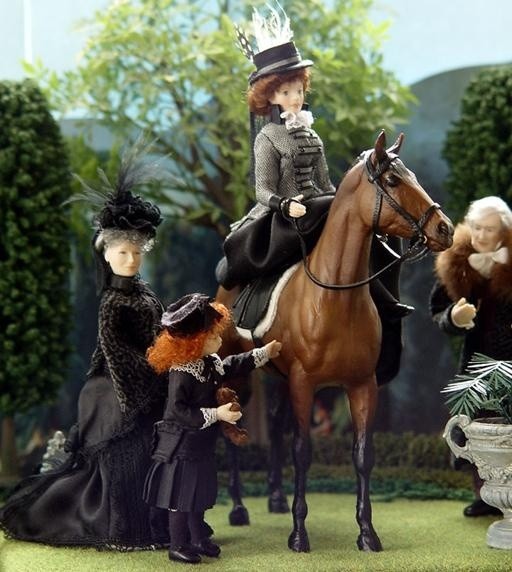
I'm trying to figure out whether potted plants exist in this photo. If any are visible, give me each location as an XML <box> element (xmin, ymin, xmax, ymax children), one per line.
<box><xmin>442</xmin><ymin>351</ymin><xmax>512</xmax><ymax>549</ymax></box>
